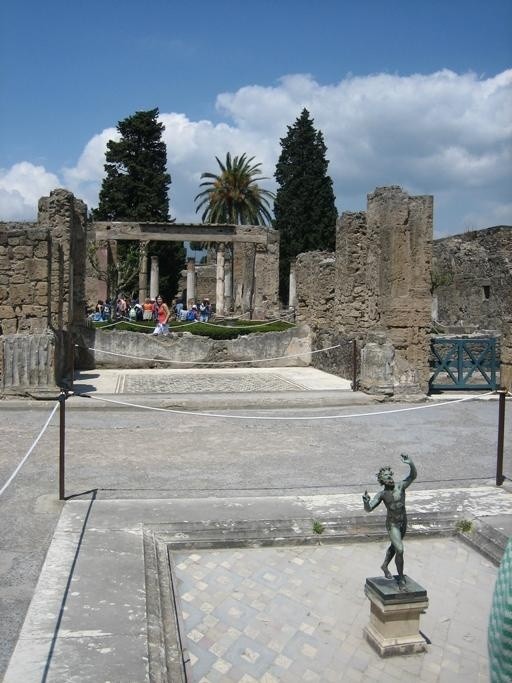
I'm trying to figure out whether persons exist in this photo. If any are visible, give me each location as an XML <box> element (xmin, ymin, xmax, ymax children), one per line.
<box><xmin>361</xmin><ymin>451</ymin><xmax>419</xmax><ymax>592</ymax></box>
<box><xmin>91</xmin><ymin>294</ymin><xmax>215</xmax><ymax>335</ymax></box>
